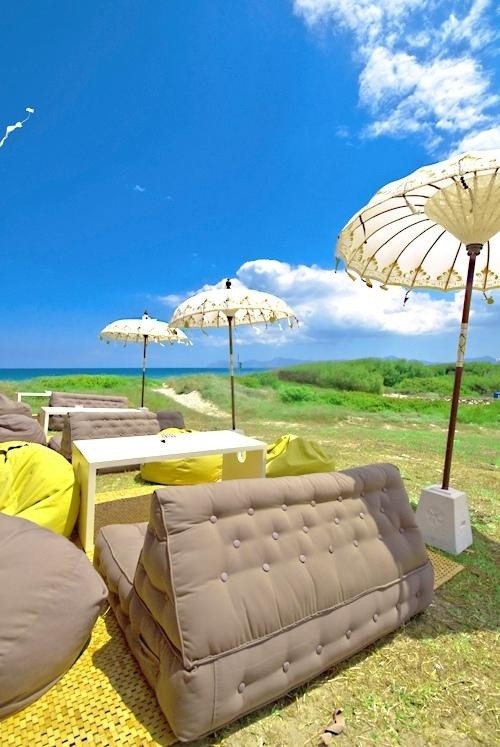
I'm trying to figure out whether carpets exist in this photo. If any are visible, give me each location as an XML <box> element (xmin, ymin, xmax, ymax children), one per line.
<box><xmin>0</xmin><ymin>483</ymin><xmax>465</xmax><ymax>747</ymax></box>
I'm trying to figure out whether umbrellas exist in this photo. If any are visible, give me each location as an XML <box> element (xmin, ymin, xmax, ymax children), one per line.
<box><xmin>337</xmin><ymin>149</ymin><xmax>499</xmax><ymax>490</ymax></box>
<box><xmin>168</xmin><ymin>278</ymin><xmax>298</xmax><ymax>430</ymax></box>
<box><xmin>98</xmin><ymin>309</ymin><xmax>191</xmax><ymax>407</ymax></box>
